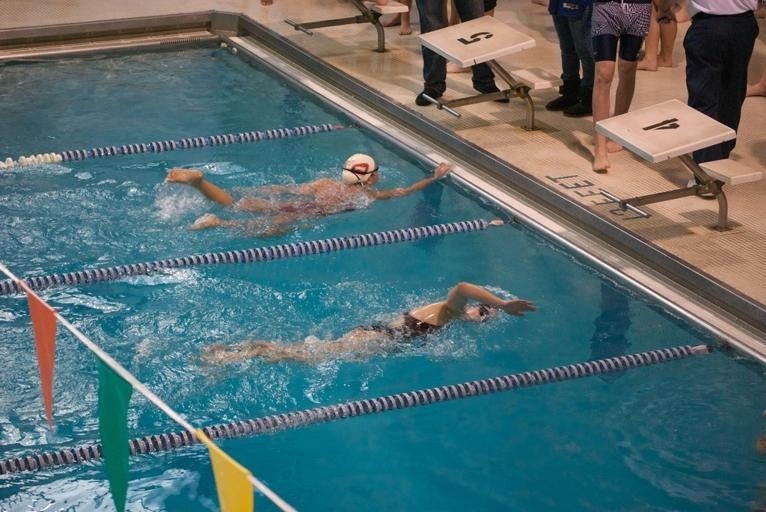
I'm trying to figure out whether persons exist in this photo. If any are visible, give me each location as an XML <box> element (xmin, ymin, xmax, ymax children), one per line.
<box><xmin>591</xmin><ymin>0</ymin><xmax>654</xmax><ymax>174</ymax></box>
<box><xmin>202</xmin><ymin>281</ymin><xmax>538</xmax><ymax>365</ymax></box>
<box><xmin>683</xmin><ymin>0</ymin><xmax>762</xmax><ymax>197</ymax></box>
<box><xmin>383</xmin><ymin>0</ymin><xmax>675</xmax><ymax>118</ymax></box>
<box><xmin>166</xmin><ymin>152</ymin><xmax>452</xmax><ymax>237</ymax></box>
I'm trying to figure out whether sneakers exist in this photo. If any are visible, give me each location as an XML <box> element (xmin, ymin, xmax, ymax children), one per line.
<box><xmin>688</xmin><ymin>180</ymin><xmax>715</xmax><ymax>196</ymax></box>
<box><xmin>416</xmin><ymin>89</ymin><xmax>437</xmax><ymax>105</ymax></box>
<box><xmin>473</xmin><ymin>84</ymin><xmax>510</xmax><ymax>102</ymax></box>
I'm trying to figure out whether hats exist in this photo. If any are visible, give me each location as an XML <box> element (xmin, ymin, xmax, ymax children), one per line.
<box><xmin>342</xmin><ymin>154</ymin><xmax>375</xmax><ymax>185</ymax></box>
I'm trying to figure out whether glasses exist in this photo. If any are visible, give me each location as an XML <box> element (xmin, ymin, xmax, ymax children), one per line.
<box><xmin>478</xmin><ymin>306</ymin><xmax>490</xmax><ymax>323</ymax></box>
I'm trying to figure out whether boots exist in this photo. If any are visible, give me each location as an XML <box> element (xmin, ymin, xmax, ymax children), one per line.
<box><xmin>546</xmin><ymin>79</ymin><xmax>593</xmax><ymax>117</ymax></box>
